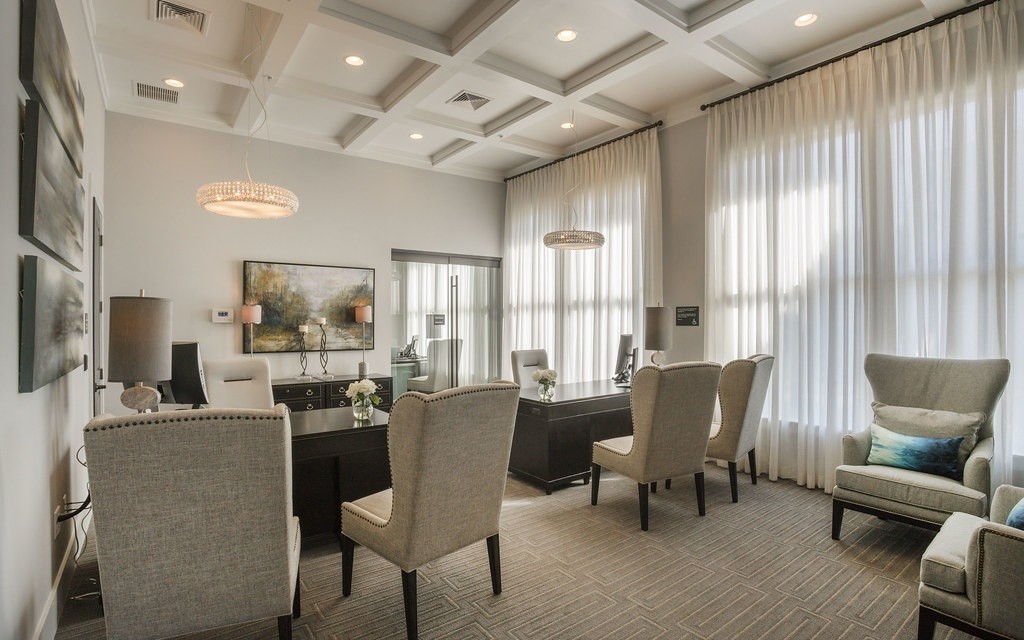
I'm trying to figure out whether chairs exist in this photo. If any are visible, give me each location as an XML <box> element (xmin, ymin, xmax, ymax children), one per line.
<box><xmin>84</xmin><ymin>339</ymin><xmax>1024</xmax><ymax>640</ymax></box>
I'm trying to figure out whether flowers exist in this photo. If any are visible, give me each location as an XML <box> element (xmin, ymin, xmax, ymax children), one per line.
<box><xmin>346</xmin><ymin>379</ymin><xmax>383</xmax><ymax>406</ymax></box>
<box><xmin>533</xmin><ymin>369</ymin><xmax>557</xmax><ymax>391</ymax></box>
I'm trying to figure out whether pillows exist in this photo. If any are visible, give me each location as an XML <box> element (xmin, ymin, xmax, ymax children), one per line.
<box><xmin>1006</xmin><ymin>497</ymin><xmax>1024</xmax><ymax>531</ymax></box>
<box><xmin>865</xmin><ymin>423</ymin><xmax>964</xmax><ymax>480</ymax></box>
<box><xmin>871</xmin><ymin>401</ymin><xmax>988</xmax><ymax>467</ymax></box>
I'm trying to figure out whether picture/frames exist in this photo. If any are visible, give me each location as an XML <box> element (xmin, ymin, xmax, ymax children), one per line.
<box><xmin>243</xmin><ymin>260</ymin><xmax>375</xmax><ymax>354</ymax></box>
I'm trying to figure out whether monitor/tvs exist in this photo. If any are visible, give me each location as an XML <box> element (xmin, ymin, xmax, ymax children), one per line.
<box><xmin>615</xmin><ymin>335</ymin><xmax>638</xmax><ymax>388</ymax></box>
<box><xmin>123</xmin><ymin>341</ymin><xmax>209</xmax><ymax>404</ymax></box>
<box><xmin>409</xmin><ymin>334</ymin><xmax>420</xmax><ymax>358</ymax></box>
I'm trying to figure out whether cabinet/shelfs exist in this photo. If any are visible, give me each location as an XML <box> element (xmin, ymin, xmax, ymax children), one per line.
<box><xmin>314</xmin><ymin>373</ymin><xmax>393</xmax><ymax>410</ymax></box>
<box><xmin>271</xmin><ymin>376</ymin><xmax>325</xmax><ymax>412</ymax></box>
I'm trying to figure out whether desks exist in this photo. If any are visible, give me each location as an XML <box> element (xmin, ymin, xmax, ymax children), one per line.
<box><xmin>392</xmin><ymin>356</ymin><xmax>427</xmax><ymax>377</ymax></box>
<box><xmin>507</xmin><ymin>380</ymin><xmax>634</xmax><ymax>495</ymax></box>
<box><xmin>290</xmin><ymin>406</ymin><xmax>390</xmax><ymax>536</ymax></box>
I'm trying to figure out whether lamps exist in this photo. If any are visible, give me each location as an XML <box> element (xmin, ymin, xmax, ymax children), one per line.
<box><xmin>355</xmin><ymin>306</ymin><xmax>373</xmax><ymax>375</ymax></box>
<box><xmin>543</xmin><ymin>108</ymin><xmax>605</xmax><ymax>250</ymax></box>
<box><xmin>645</xmin><ymin>302</ymin><xmax>672</xmax><ymax>367</ymax></box>
<box><xmin>107</xmin><ymin>288</ymin><xmax>172</xmax><ymax>414</ymax></box>
<box><xmin>242</xmin><ymin>305</ymin><xmax>262</xmax><ymax>357</ymax></box>
<box><xmin>195</xmin><ymin>0</ymin><xmax>299</xmax><ymax>218</ymax></box>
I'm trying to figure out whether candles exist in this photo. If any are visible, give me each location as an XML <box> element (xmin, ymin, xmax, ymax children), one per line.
<box><xmin>299</xmin><ymin>326</ymin><xmax>308</xmax><ymax>333</ymax></box>
<box><xmin>317</xmin><ymin>317</ymin><xmax>326</xmax><ymax>325</ymax></box>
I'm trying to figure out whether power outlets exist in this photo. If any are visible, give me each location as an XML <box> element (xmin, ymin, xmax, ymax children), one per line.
<box><xmin>53</xmin><ymin>504</ymin><xmax>63</xmax><ymax>540</ymax></box>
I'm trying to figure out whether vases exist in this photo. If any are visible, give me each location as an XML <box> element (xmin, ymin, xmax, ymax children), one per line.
<box><xmin>538</xmin><ymin>385</ymin><xmax>554</xmax><ymax>399</ymax></box>
<box><xmin>352</xmin><ymin>398</ymin><xmax>373</xmax><ymax>419</ymax></box>
